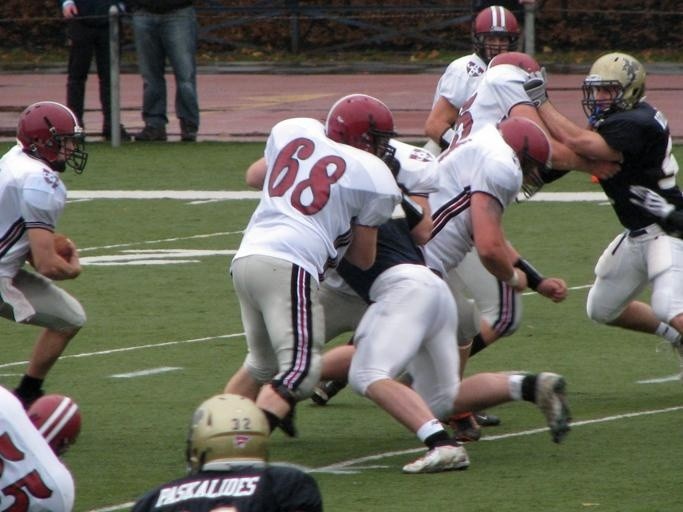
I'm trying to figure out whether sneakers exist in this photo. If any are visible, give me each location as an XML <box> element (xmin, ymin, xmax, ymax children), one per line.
<box><xmin>537</xmin><ymin>371</ymin><xmax>571</xmax><ymax>444</ymax></box>
<box><xmin>403</xmin><ymin>411</ymin><xmax>502</xmax><ymax>473</ymax></box>
<box><xmin>310</xmin><ymin>379</ymin><xmax>345</xmax><ymax>405</ymax></box>
<box><xmin>102</xmin><ymin>124</ymin><xmax>197</xmax><ymax>141</ymax></box>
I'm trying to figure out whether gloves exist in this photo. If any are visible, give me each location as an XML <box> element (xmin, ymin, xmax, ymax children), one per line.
<box><xmin>632</xmin><ymin>183</ymin><xmax>675</xmax><ymax>223</ymax></box>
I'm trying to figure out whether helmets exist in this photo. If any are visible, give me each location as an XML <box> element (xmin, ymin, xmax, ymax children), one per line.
<box><xmin>326</xmin><ymin>95</ymin><xmax>397</xmax><ymax>166</ymax></box>
<box><xmin>17</xmin><ymin>102</ymin><xmax>87</xmax><ymax>174</ymax></box>
<box><xmin>471</xmin><ymin>5</ymin><xmax>552</xmax><ymax>203</ymax></box>
<box><xmin>580</xmin><ymin>51</ymin><xmax>647</xmax><ymax>123</ymax></box>
<box><xmin>27</xmin><ymin>396</ymin><xmax>81</xmax><ymax>456</ymax></box>
<box><xmin>186</xmin><ymin>394</ymin><xmax>271</xmax><ymax>469</ymax></box>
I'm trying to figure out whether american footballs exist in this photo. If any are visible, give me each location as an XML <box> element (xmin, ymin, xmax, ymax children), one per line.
<box><xmin>27</xmin><ymin>232</ymin><xmax>73</xmax><ymax>269</ymax></box>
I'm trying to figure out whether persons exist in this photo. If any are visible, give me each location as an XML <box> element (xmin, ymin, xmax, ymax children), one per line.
<box><xmin>424</xmin><ymin>6</ymin><xmax>683</xmax><ymax>354</ymax></box>
<box><xmin>0</xmin><ymin>384</ymin><xmax>82</xmax><ymax>512</ymax></box>
<box><xmin>60</xmin><ymin>0</ymin><xmax>129</xmax><ymax>142</ymax></box>
<box><xmin>131</xmin><ymin>393</ymin><xmax>323</xmax><ymax>512</ymax></box>
<box><xmin>221</xmin><ymin>92</ymin><xmax>573</xmax><ymax>475</ymax></box>
<box><xmin>124</xmin><ymin>0</ymin><xmax>200</xmax><ymax>142</ymax></box>
<box><xmin>0</xmin><ymin>101</ymin><xmax>87</xmax><ymax>411</ymax></box>
